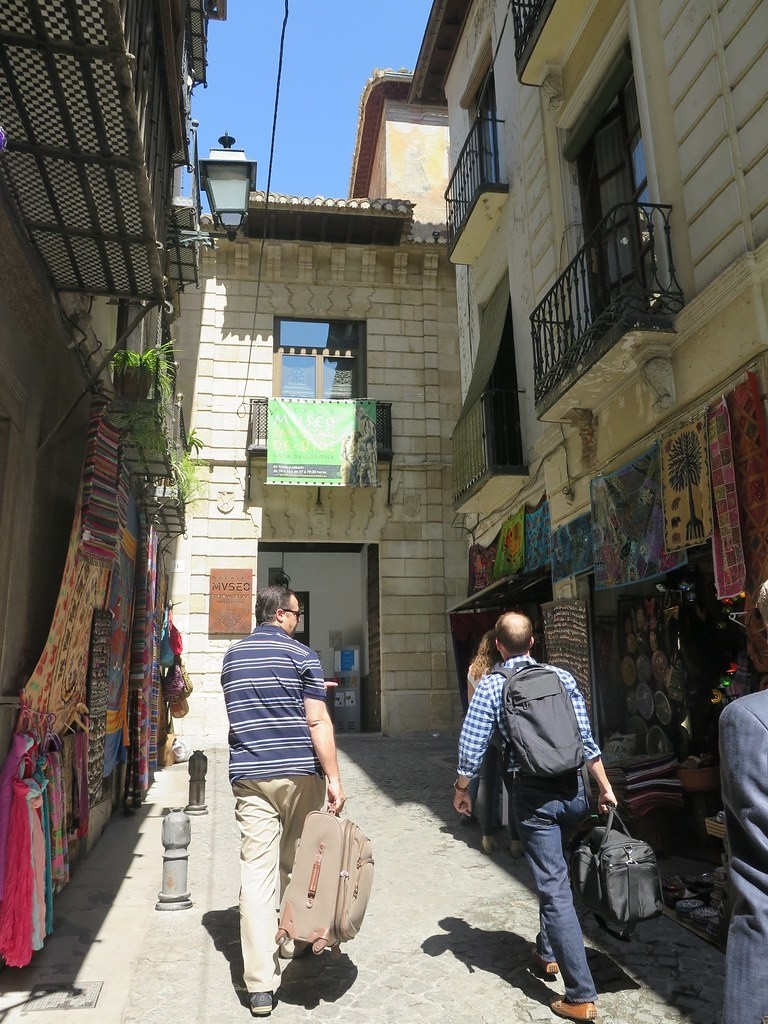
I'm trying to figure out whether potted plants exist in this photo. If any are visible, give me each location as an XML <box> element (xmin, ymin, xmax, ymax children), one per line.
<box><xmin>111</xmin><ymin>338</ymin><xmax>181</xmax><ymax>401</ymax></box>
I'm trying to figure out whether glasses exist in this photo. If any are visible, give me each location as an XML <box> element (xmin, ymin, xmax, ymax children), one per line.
<box><xmin>281</xmin><ymin>609</ymin><xmax>301</xmax><ymax>618</ymax></box>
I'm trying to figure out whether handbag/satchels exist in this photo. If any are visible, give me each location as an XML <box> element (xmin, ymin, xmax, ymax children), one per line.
<box><xmin>161</xmin><ymin>606</ymin><xmax>193</xmax><ymax>717</ymax></box>
<box><xmin>569</xmin><ymin>803</ymin><xmax>664</xmax><ymax>943</ymax></box>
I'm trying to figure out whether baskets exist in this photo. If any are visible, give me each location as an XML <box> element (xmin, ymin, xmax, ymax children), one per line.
<box><xmin>705</xmin><ymin>817</ymin><xmax>727</xmax><ymax>839</ymax></box>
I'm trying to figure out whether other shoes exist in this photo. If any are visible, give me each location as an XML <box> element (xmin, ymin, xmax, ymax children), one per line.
<box><xmin>245</xmin><ymin>990</ymin><xmax>273</xmax><ymax>1014</ymax></box>
<box><xmin>550</xmin><ymin>994</ymin><xmax>597</xmax><ymax>1020</ymax></box>
<box><xmin>531</xmin><ymin>948</ymin><xmax>559</xmax><ymax>975</ymax></box>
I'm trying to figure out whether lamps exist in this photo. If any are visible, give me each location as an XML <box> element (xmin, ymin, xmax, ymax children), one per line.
<box><xmin>168</xmin><ymin>130</ymin><xmax>257</xmax><ymax>239</ymax></box>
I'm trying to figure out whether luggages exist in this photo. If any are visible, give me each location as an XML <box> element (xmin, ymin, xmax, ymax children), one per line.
<box><xmin>275</xmin><ymin>806</ymin><xmax>374</xmax><ymax>959</ymax></box>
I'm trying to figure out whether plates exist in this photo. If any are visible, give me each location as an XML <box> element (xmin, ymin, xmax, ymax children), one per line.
<box><xmin>619</xmin><ymin>599</ymin><xmax>674</xmax><ymax>758</ymax></box>
<box><xmin>661</xmin><ymin>865</ymin><xmax>725</xmax><ymax>937</ymax></box>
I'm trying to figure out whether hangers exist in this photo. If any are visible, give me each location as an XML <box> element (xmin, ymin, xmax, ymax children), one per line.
<box><xmin>15</xmin><ymin>706</ymin><xmax>89</xmax><ymax>779</ymax></box>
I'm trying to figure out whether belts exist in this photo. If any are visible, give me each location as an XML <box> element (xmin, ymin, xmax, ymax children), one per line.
<box><xmin>511</xmin><ymin>769</ymin><xmax>582</xmax><ymax>778</ymax></box>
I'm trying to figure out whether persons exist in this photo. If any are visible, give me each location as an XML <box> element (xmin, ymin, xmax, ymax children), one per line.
<box><xmin>454</xmin><ymin>612</ymin><xmax>617</xmax><ymax>1021</ymax></box>
<box><xmin>718</xmin><ymin>581</ymin><xmax>768</xmax><ymax>1024</ymax></box>
<box><xmin>468</xmin><ymin>631</ymin><xmax>524</xmax><ymax>857</ymax></box>
<box><xmin>221</xmin><ymin>586</ymin><xmax>346</xmax><ymax>1014</ymax></box>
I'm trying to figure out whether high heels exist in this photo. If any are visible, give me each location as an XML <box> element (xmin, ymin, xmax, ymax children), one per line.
<box><xmin>510</xmin><ymin>839</ymin><xmax>525</xmax><ymax>857</ymax></box>
<box><xmin>481</xmin><ymin>834</ymin><xmax>499</xmax><ymax>854</ymax></box>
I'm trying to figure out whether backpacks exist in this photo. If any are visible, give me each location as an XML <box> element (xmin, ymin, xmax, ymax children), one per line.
<box><xmin>489</xmin><ymin>663</ymin><xmax>585</xmax><ymax>786</ymax></box>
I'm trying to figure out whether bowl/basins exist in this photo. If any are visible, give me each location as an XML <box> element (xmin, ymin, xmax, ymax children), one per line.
<box><xmin>674</xmin><ymin>765</ymin><xmax>721</xmax><ymax>792</ymax></box>
